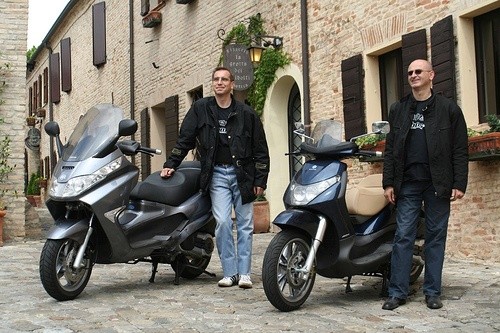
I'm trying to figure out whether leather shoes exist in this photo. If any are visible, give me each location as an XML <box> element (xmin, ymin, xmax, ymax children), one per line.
<box><xmin>425</xmin><ymin>294</ymin><xmax>442</xmax><ymax>309</ymax></box>
<box><xmin>382</xmin><ymin>296</ymin><xmax>406</xmax><ymax>310</ymax></box>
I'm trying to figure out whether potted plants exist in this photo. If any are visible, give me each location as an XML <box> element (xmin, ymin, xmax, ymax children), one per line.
<box><xmin>33</xmin><ymin>178</ymin><xmax>40</xmax><ymax>208</ymax></box>
<box><xmin>253</xmin><ymin>193</ymin><xmax>270</xmax><ymax>233</ymax></box>
<box><xmin>0</xmin><ymin>135</ymin><xmax>18</xmax><ymax>247</ymax></box>
<box><xmin>26</xmin><ymin>173</ymin><xmax>35</xmax><ymax>204</ymax></box>
<box><xmin>467</xmin><ymin>114</ymin><xmax>500</xmax><ymax>161</ymax></box>
<box><xmin>354</xmin><ymin>134</ymin><xmax>385</xmax><ymax>162</ymax></box>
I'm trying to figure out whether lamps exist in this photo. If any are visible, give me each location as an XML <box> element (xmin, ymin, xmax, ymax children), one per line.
<box><xmin>217</xmin><ymin>28</ymin><xmax>284</xmax><ymax>63</ymax></box>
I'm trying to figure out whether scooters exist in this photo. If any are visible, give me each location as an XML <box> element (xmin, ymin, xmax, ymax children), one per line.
<box><xmin>37</xmin><ymin>102</ymin><xmax>217</xmax><ymax>302</ymax></box>
<box><xmin>261</xmin><ymin>117</ymin><xmax>427</xmax><ymax>312</ymax></box>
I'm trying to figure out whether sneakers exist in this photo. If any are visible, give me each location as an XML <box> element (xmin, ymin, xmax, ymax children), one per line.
<box><xmin>217</xmin><ymin>274</ymin><xmax>239</xmax><ymax>286</ymax></box>
<box><xmin>239</xmin><ymin>274</ymin><xmax>253</xmax><ymax>288</ymax></box>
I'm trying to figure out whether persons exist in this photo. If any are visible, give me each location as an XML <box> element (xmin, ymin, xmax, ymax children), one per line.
<box><xmin>382</xmin><ymin>59</ymin><xmax>469</xmax><ymax>310</ymax></box>
<box><xmin>160</xmin><ymin>67</ymin><xmax>269</xmax><ymax>288</ymax></box>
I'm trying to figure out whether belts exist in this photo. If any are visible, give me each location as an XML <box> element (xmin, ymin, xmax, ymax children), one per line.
<box><xmin>214</xmin><ymin>161</ymin><xmax>234</xmax><ymax>169</ymax></box>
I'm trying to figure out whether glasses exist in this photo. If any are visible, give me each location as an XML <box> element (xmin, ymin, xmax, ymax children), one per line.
<box><xmin>407</xmin><ymin>69</ymin><xmax>431</xmax><ymax>75</ymax></box>
